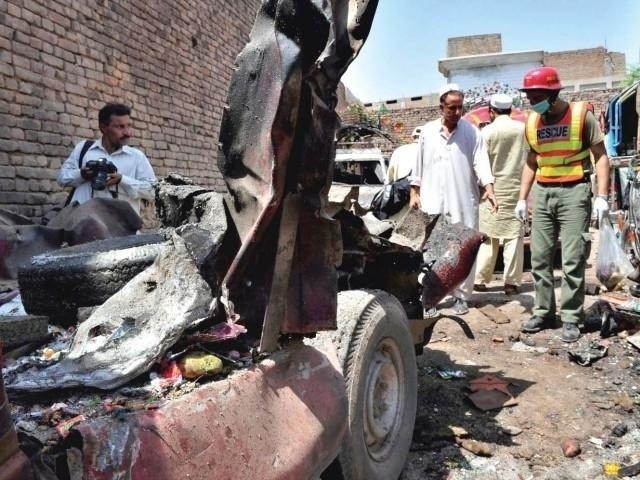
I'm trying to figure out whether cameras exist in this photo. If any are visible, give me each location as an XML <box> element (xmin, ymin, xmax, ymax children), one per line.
<box><xmin>86</xmin><ymin>157</ymin><xmax>119</xmax><ymax>191</ymax></box>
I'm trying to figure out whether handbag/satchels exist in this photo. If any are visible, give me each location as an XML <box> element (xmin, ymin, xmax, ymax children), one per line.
<box><xmin>370</xmin><ymin>178</ymin><xmax>411</xmax><ymax>219</ymax></box>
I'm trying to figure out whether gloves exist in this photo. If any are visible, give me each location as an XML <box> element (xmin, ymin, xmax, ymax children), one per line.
<box><xmin>594</xmin><ymin>193</ymin><xmax>609</xmax><ymax>222</ymax></box>
<box><xmin>514</xmin><ymin>199</ymin><xmax>528</xmax><ymax>224</ymax></box>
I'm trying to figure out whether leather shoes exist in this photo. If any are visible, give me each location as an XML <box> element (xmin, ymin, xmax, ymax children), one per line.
<box><xmin>562</xmin><ymin>322</ymin><xmax>581</xmax><ymax>342</ymax></box>
<box><xmin>520</xmin><ymin>314</ymin><xmax>556</xmax><ymax>332</ymax></box>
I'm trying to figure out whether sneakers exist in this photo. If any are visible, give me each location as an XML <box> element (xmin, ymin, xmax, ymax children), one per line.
<box><xmin>452</xmin><ymin>296</ymin><xmax>469</xmax><ymax>316</ymax></box>
<box><xmin>503</xmin><ymin>283</ymin><xmax>518</xmax><ymax>295</ymax></box>
<box><xmin>424</xmin><ymin>307</ymin><xmax>439</xmax><ymax>319</ymax></box>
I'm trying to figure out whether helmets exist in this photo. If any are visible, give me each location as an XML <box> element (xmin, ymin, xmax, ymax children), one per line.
<box><xmin>518</xmin><ymin>67</ymin><xmax>564</xmax><ymax>90</ymax></box>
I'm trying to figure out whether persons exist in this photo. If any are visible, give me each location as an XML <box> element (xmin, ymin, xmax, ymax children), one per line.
<box><xmin>474</xmin><ymin>95</ymin><xmax>531</xmax><ymax>295</ymax></box>
<box><xmin>383</xmin><ymin>125</ymin><xmax>423</xmax><ymax>225</ymax></box>
<box><xmin>409</xmin><ymin>84</ymin><xmax>499</xmax><ymax>316</ymax></box>
<box><xmin>58</xmin><ymin>101</ymin><xmax>158</xmax><ymax>218</ymax></box>
<box><xmin>513</xmin><ymin>67</ymin><xmax>611</xmax><ymax>342</ymax></box>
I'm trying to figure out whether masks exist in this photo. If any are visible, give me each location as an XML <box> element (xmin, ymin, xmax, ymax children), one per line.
<box><xmin>528</xmin><ymin>97</ymin><xmax>551</xmax><ymax>115</ymax></box>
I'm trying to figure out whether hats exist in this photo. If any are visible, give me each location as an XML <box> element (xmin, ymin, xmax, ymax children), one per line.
<box><xmin>411</xmin><ymin>126</ymin><xmax>422</xmax><ymax>137</ymax></box>
<box><xmin>439</xmin><ymin>83</ymin><xmax>464</xmax><ymax>103</ymax></box>
<box><xmin>490</xmin><ymin>93</ymin><xmax>513</xmax><ymax>110</ymax></box>
